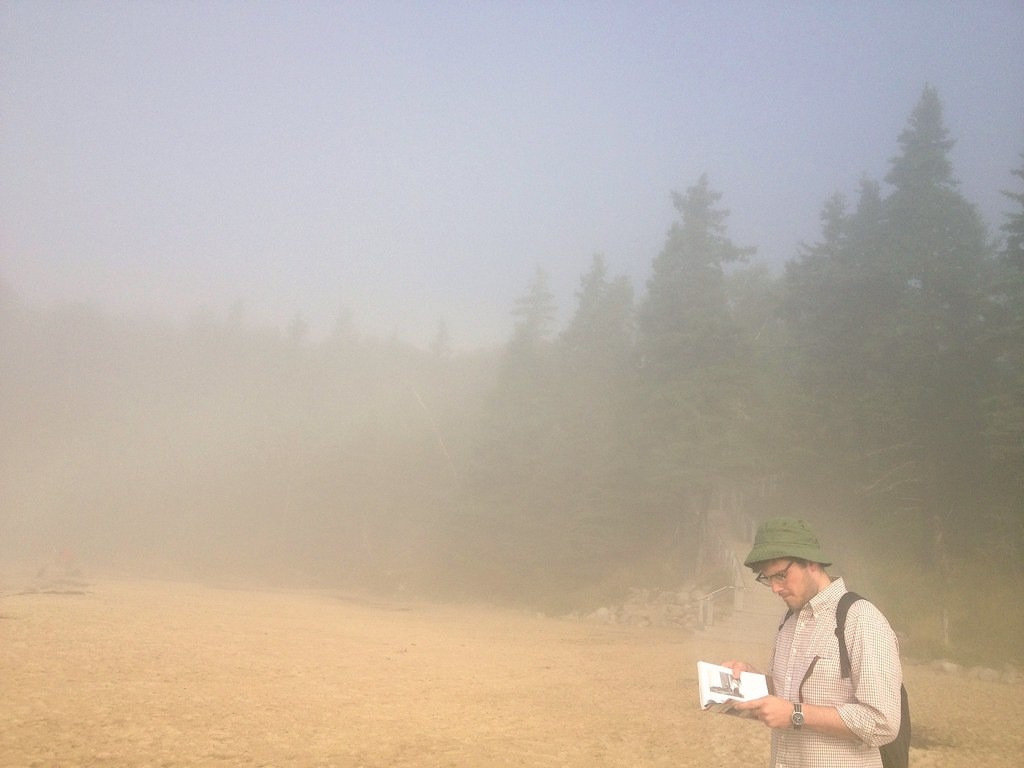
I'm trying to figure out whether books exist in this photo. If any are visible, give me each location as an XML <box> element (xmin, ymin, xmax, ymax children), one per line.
<box><xmin>697</xmin><ymin>661</ymin><xmax>769</xmax><ymax>720</ymax></box>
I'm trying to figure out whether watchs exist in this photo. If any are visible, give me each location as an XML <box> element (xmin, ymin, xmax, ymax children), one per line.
<box><xmin>792</xmin><ymin>702</ymin><xmax>803</xmax><ymax>731</ymax></box>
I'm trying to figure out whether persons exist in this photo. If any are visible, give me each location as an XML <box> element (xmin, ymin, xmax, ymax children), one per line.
<box><xmin>720</xmin><ymin>518</ymin><xmax>903</xmax><ymax>768</ymax></box>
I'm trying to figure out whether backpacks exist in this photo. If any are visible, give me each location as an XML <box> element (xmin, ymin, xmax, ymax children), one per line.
<box><xmin>779</xmin><ymin>592</ymin><xmax>911</xmax><ymax>768</ymax></box>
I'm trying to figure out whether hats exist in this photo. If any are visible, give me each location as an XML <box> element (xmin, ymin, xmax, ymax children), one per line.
<box><xmin>744</xmin><ymin>517</ymin><xmax>833</xmax><ymax>567</ymax></box>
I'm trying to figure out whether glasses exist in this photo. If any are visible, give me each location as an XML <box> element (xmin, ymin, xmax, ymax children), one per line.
<box><xmin>755</xmin><ymin>558</ymin><xmax>794</xmax><ymax>587</ymax></box>
